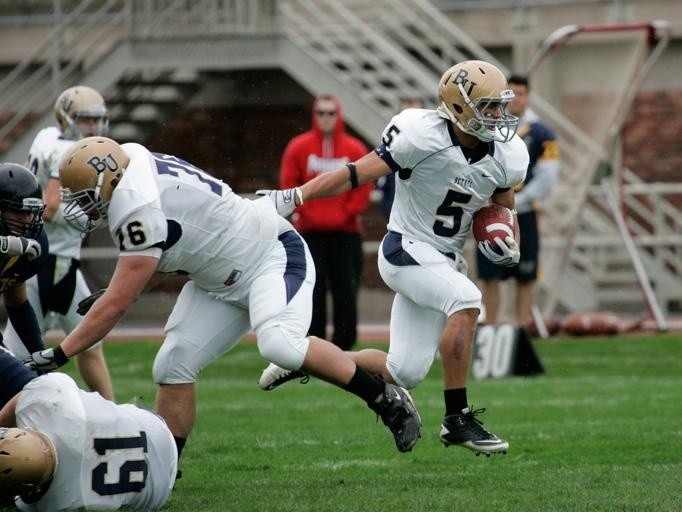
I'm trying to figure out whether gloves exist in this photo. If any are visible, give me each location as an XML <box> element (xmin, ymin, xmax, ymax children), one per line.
<box><xmin>76</xmin><ymin>286</ymin><xmax>109</xmax><ymax>316</ymax></box>
<box><xmin>19</xmin><ymin>344</ymin><xmax>70</xmax><ymax>376</ymax></box>
<box><xmin>255</xmin><ymin>183</ymin><xmax>303</xmax><ymax>217</ymax></box>
<box><xmin>0</xmin><ymin>235</ymin><xmax>42</xmax><ymax>261</ymax></box>
<box><xmin>475</xmin><ymin>237</ymin><xmax>524</xmax><ymax>269</ymax></box>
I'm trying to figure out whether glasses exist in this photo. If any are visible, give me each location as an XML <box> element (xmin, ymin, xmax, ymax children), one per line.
<box><xmin>315</xmin><ymin>109</ymin><xmax>337</xmax><ymax>117</ymax></box>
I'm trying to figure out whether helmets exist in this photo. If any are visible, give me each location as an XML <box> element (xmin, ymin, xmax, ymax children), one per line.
<box><xmin>0</xmin><ymin>425</ymin><xmax>56</xmax><ymax>499</ymax></box>
<box><xmin>50</xmin><ymin>84</ymin><xmax>113</xmax><ymax>142</ymax></box>
<box><xmin>0</xmin><ymin>160</ymin><xmax>47</xmax><ymax>244</ymax></box>
<box><xmin>56</xmin><ymin>133</ymin><xmax>131</xmax><ymax>237</ymax></box>
<box><xmin>436</xmin><ymin>58</ymin><xmax>520</xmax><ymax>147</ymax></box>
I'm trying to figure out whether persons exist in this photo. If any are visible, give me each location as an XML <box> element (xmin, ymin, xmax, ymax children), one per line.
<box><xmin>473</xmin><ymin>74</ymin><xmax>560</xmax><ymax>331</ymax></box>
<box><xmin>0</xmin><ymin>85</ymin><xmax>117</xmax><ymax>403</ymax></box>
<box><xmin>275</xmin><ymin>91</ymin><xmax>376</xmax><ymax>357</ymax></box>
<box><xmin>1</xmin><ymin>162</ymin><xmax>53</xmax><ymax>408</ymax></box>
<box><xmin>1</xmin><ymin>370</ymin><xmax>180</xmax><ymax>510</ymax></box>
<box><xmin>13</xmin><ymin>137</ymin><xmax>423</xmax><ymax>490</ymax></box>
<box><xmin>253</xmin><ymin>58</ymin><xmax>530</xmax><ymax>459</ymax></box>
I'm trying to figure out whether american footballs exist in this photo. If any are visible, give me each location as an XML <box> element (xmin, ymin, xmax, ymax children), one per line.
<box><xmin>560</xmin><ymin>313</ymin><xmax>617</xmax><ymax>334</ymax></box>
<box><xmin>473</xmin><ymin>203</ymin><xmax>516</xmax><ymax>254</ymax></box>
<box><xmin>526</xmin><ymin>317</ymin><xmax>560</xmax><ymax>338</ymax></box>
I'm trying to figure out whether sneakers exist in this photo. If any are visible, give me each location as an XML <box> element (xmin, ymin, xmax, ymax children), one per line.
<box><xmin>436</xmin><ymin>409</ymin><xmax>514</xmax><ymax>458</ymax></box>
<box><xmin>255</xmin><ymin>360</ymin><xmax>307</xmax><ymax>392</ymax></box>
<box><xmin>383</xmin><ymin>380</ymin><xmax>426</xmax><ymax>455</ymax></box>
